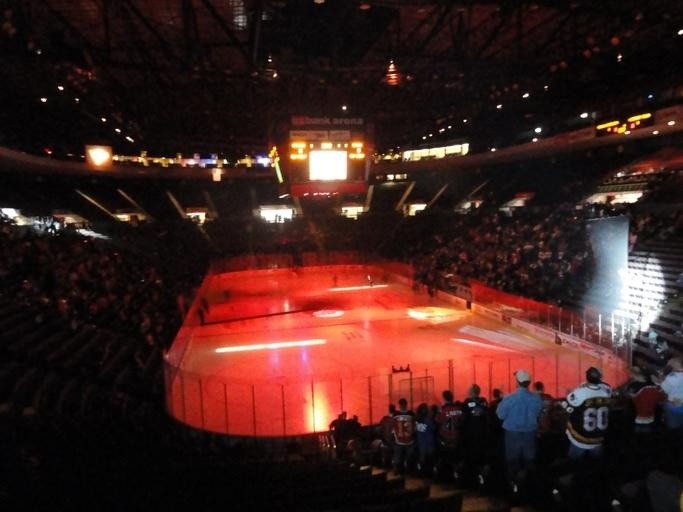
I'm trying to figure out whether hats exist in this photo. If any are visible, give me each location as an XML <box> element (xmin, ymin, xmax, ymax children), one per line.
<box><xmin>513</xmin><ymin>370</ymin><xmax>530</xmax><ymax>383</ymax></box>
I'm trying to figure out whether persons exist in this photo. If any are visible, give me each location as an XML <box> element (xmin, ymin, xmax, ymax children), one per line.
<box><xmin>327</xmin><ymin>357</ymin><xmax>682</xmax><ymax>512</ymax></box>
<box><xmin>0</xmin><ymin>205</ymin><xmax>202</xmax><ymax>512</ymax></box>
<box><xmin>646</xmin><ymin>321</ymin><xmax>683</xmax><ymax>360</ymax></box>
<box><xmin>202</xmin><ymin>175</ymin><xmax>683</xmax><ymax>307</ymax></box>
